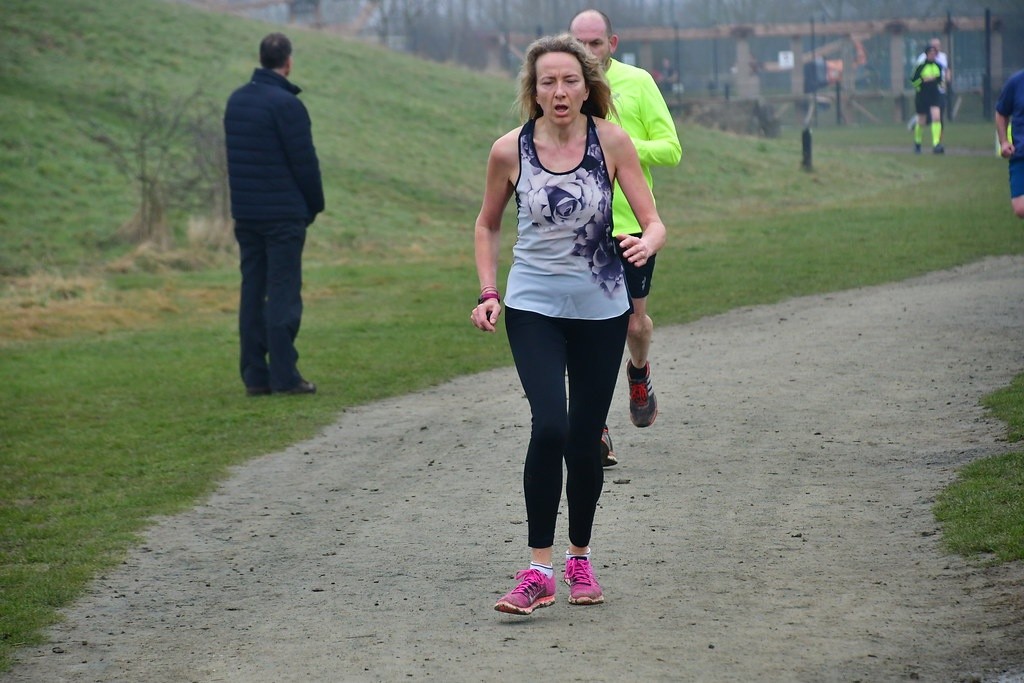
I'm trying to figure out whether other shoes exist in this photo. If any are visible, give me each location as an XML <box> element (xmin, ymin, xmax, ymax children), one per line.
<box><xmin>274</xmin><ymin>380</ymin><xmax>316</xmax><ymax>397</ymax></box>
<box><xmin>247</xmin><ymin>382</ymin><xmax>274</xmax><ymax>397</ymax></box>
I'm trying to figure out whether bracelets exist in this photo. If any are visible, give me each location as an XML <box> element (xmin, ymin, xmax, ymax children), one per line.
<box><xmin>481</xmin><ymin>285</ymin><xmax>500</xmax><ymax>295</ymax></box>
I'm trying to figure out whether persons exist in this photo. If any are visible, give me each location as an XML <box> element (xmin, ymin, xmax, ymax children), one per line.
<box><xmin>906</xmin><ymin>38</ymin><xmax>951</xmax><ymax>152</ymax></box>
<box><xmin>651</xmin><ymin>57</ymin><xmax>677</xmax><ymax>93</ymax></box>
<box><xmin>568</xmin><ymin>9</ymin><xmax>682</xmax><ymax>468</ymax></box>
<box><xmin>471</xmin><ymin>31</ymin><xmax>664</xmax><ymax>617</ymax></box>
<box><xmin>994</xmin><ymin>69</ymin><xmax>1024</xmax><ymax>221</ymax></box>
<box><xmin>220</xmin><ymin>31</ymin><xmax>325</xmax><ymax>395</ymax></box>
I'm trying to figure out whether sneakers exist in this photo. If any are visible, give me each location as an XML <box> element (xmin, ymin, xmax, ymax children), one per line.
<box><xmin>494</xmin><ymin>569</ymin><xmax>556</xmax><ymax>615</ymax></box>
<box><xmin>626</xmin><ymin>361</ymin><xmax>657</xmax><ymax>430</ymax></box>
<box><xmin>564</xmin><ymin>553</ymin><xmax>605</xmax><ymax>605</ymax></box>
<box><xmin>594</xmin><ymin>423</ymin><xmax>618</xmax><ymax>469</ymax></box>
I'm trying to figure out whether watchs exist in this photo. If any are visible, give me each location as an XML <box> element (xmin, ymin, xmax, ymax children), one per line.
<box><xmin>477</xmin><ymin>292</ymin><xmax>500</xmax><ymax>305</ymax></box>
